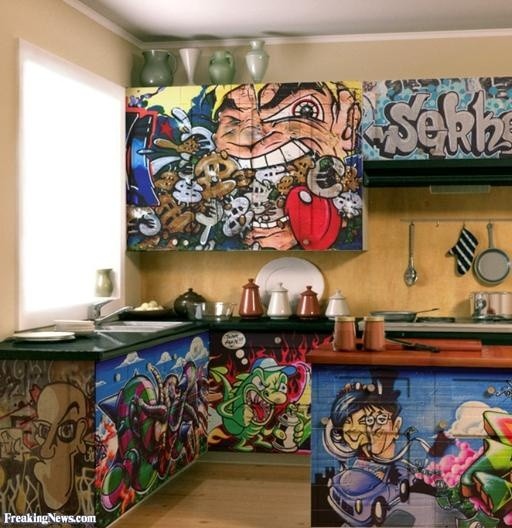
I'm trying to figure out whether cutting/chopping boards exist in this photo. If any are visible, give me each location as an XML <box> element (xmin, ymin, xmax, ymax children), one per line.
<box><xmin>336</xmin><ymin>339</ymin><xmax>483</xmax><ymax>352</ymax></box>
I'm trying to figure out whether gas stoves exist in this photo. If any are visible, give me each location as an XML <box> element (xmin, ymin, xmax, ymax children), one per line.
<box><xmin>385</xmin><ymin>310</ymin><xmax>512</xmax><ymax>326</ymax></box>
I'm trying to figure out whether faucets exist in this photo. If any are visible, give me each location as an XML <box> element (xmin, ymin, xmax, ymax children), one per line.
<box><xmin>88</xmin><ymin>297</ymin><xmax>133</xmax><ymax>330</ymax></box>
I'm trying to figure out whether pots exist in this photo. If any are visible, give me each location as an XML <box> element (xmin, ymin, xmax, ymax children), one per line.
<box><xmin>470</xmin><ymin>290</ymin><xmax>512</xmax><ymax>323</ymax></box>
<box><xmin>473</xmin><ymin>223</ymin><xmax>510</xmax><ymax>285</ymax></box>
<box><xmin>371</xmin><ymin>306</ymin><xmax>442</xmax><ymax>322</ymax></box>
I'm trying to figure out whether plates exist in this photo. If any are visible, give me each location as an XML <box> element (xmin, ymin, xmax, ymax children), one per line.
<box><xmin>55</xmin><ymin>320</ymin><xmax>95</xmax><ymax>337</ymax></box>
<box><xmin>12</xmin><ymin>332</ymin><xmax>76</xmax><ymax>342</ymax></box>
<box><xmin>255</xmin><ymin>256</ymin><xmax>325</xmax><ymax>315</ymax></box>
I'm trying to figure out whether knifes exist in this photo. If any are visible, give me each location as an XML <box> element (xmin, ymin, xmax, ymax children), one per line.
<box><xmin>387</xmin><ymin>336</ymin><xmax>442</xmax><ymax>354</ymax></box>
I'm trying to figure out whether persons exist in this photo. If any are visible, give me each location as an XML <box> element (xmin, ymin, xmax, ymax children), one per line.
<box><xmin>213</xmin><ymin>83</ymin><xmax>360</xmax><ymax>251</ymax></box>
<box><xmin>322</xmin><ymin>389</ymin><xmax>378</xmax><ymax>471</ymax></box>
<box><xmin>367</xmin><ymin>396</ymin><xmax>402</xmax><ymax>462</ymax></box>
<box><xmin>32</xmin><ymin>382</ymin><xmax>88</xmax><ymax>527</ymax></box>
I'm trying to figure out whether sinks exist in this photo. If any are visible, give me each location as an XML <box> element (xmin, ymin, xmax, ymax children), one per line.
<box><xmin>96</xmin><ymin>321</ymin><xmax>193</xmax><ymax>334</ymax></box>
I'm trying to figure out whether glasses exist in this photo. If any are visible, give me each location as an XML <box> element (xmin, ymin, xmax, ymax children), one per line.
<box><xmin>365</xmin><ymin>413</ymin><xmax>393</xmax><ymax>426</ymax></box>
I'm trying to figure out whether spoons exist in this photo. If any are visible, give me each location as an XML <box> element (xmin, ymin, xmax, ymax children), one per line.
<box><xmin>403</xmin><ymin>224</ymin><xmax>417</xmax><ymax>287</ymax></box>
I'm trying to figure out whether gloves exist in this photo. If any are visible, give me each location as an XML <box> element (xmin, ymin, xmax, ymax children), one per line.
<box><xmin>448</xmin><ymin>228</ymin><xmax>479</xmax><ymax>276</ymax></box>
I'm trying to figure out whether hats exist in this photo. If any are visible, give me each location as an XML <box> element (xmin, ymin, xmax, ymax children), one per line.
<box><xmin>252</xmin><ymin>358</ymin><xmax>297</xmax><ymax>378</ymax></box>
<box><xmin>356</xmin><ymin>361</ymin><xmax>402</xmax><ymax>405</ymax></box>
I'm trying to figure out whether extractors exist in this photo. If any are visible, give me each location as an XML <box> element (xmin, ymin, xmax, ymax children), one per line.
<box><xmin>362</xmin><ymin>76</ymin><xmax>512</xmax><ymax>188</ymax></box>
